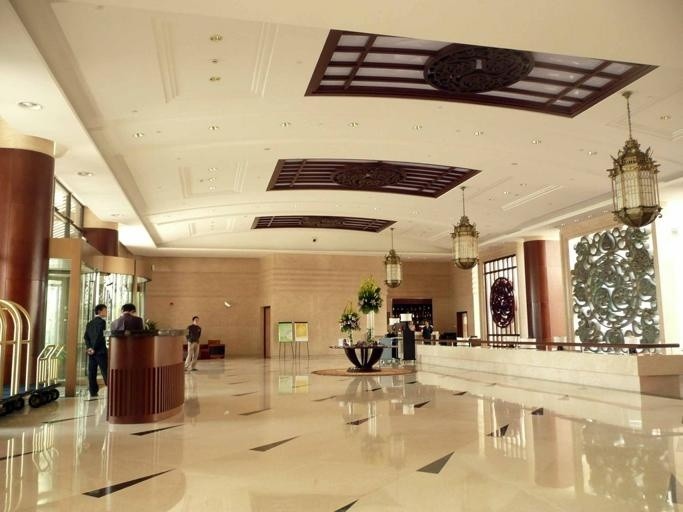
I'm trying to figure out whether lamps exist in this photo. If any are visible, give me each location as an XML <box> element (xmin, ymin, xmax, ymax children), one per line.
<box><xmin>383</xmin><ymin>227</ymin><xmax>403</xmax><ymax>289</ymax></box>
<box><xmin>607</xmin><ymin>89</ymin><xmax>663</xmax><ymax>230</ymax></box>
<box><xmin>448</xmin><ymin>186</ymin><xmax>480</xmax><ymax>270</ymax></box>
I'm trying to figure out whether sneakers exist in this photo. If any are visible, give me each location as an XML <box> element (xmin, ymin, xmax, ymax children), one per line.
<box><xmin>87</xmin><ymin>394</ymin><xmax>104</xmax><ymax>402</ymax></box>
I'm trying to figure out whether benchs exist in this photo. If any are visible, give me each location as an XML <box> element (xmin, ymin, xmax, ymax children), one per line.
<box><xmin>183</xmin><ymin>339</ymin><xmax>225</xmax><ymax>360</ymax></box>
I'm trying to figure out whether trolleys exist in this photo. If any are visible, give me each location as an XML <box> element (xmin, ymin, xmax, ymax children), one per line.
<box><xmin>0</xmin><ymin>300</ymin><xmax>33</xmax><ymax>414</ymax></box>
<box><xmin>0</xmin><ymin>301</ymin><xmax>17</xmax><ymax>417</ymax></box>
<box><xmin>0</xmin><ymin>344</ymin><xmax>55</xmax><ymax>409</ymax></box>
<box><xmin>4</xmin><ymin>344</ymin><xmax>65</xmax><ymax>403</ymax></box>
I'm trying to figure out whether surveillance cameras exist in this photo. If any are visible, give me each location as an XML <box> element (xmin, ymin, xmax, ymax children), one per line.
<box><xmin>313</xmin><ymin>238</ymin><xmax>316</xmax><ymax>242</ymax></box>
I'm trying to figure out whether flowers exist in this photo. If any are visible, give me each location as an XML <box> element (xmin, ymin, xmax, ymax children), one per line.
<box><xmin>355</xmin><ymin>273</ymin><xmax>383</xmax><ymax>317</ymax></box>
<box><xmin>337</xmin><ymin>299</ymin><xmax>362</xmax><ymax>345</ymax></box>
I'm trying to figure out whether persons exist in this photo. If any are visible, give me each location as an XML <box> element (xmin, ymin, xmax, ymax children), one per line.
<box><xmin>423</xmin><ymin>319</ymin><xmax>433</xmax><ymax>344</ymax></box>
<box><xmin>84</xmin><ymin>304</ymin><xmax>107</xmax><ymax>400</ymax></box>
<box><xmin>110</xmin><ymin>303</ymin><xmax>142</xmax><ymax>331</ymax></box>
<box><xmin>184</xmin><ymin>316</ymin><xmax>201</xmax><ymax>371</ymax></box>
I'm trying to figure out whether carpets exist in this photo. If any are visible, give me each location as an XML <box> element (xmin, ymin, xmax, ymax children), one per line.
<box><xmin>311</xmin><ymin>365</ymin><xmax>415</xmax><ymax>376</ymax></box>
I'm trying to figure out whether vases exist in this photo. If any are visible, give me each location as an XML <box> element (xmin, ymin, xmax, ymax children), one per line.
<box><xmin>365</xmin><ymin>311</ymin><xmax>374</xmax><ymax>342</ymax></box>
<box><xmin>347</xmin><ymin>329</ymin><xmax>354</xmax><ymax>345</ymax></box>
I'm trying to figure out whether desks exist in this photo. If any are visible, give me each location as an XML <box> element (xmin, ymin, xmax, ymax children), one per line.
<box><xmin>328</xmin><ymin>342</ymin><xmax>399</xmax><ymax>373</ymax></box>
